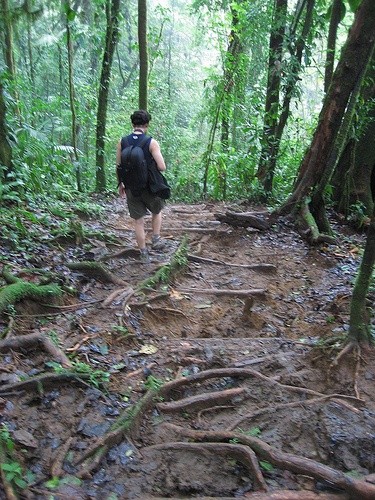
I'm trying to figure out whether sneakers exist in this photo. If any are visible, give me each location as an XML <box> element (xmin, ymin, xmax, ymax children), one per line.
<box><xmin>151</xmin><ymin>235</ymin><xmax>166</xmax><ymax>248</ymax></box>
<box><xmin>140</xmin><ymin>248</ymin><xmax>149</xmax><ymax>260</ymax></box>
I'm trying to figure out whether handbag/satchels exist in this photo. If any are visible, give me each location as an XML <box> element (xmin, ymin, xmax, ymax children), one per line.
<box><xmin>147</xmin><ymin>158</ymin><xmax>170</xmax><ymax>199</ymax></box>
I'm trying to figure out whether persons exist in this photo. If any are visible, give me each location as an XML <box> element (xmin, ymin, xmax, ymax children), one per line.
<box><xmin>116</xmin><ymin>110</ymin><xmax>166</xmax><ymax>256</ymax></box>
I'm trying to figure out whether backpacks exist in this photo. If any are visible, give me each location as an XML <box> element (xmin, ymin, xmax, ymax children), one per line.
<box><xmin>116</xmin><ymin>135</ymin><xmax>151</xmax><ymax>193</ymax></box>
